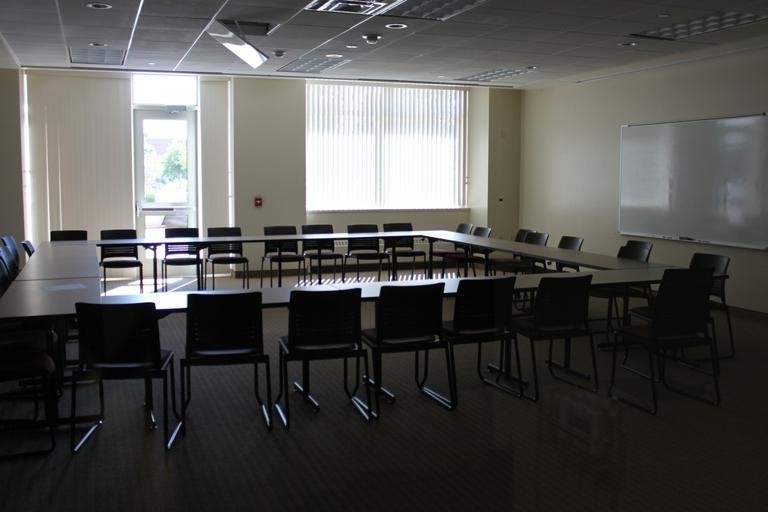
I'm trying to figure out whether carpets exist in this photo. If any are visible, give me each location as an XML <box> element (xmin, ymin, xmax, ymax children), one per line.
<box><xmin>1</xmin><ymin>266</ymin><xmax>768</xmax><ymax>511</ymax></box>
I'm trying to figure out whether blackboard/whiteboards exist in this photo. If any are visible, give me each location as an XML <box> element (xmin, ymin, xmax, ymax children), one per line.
<box><xmin>618</xmin><ymin>113</ymin><xmax>768</xmax><ymax>250</ymax></box>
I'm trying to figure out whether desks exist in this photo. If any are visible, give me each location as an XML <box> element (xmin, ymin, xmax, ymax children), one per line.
<box><xmin>0</xmin><ymin>241</ymin><xmax>106</xmax><ymax>425</ymax></box>
<box><xmin>100</xmin><ymin>281</ymin><xmax>731</xmax><ymax>431</ymax></box>
<box><xmin>81</xmin><ymin>231</ymin><xmax>456</xmax><ymax>288</ymax></box>
<box><xmin>422</xmin><ymin>229</ymin><xmax>692</xmax><ymax>413</ymax></box>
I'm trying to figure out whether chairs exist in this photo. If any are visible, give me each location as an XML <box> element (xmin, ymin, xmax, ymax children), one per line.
<box><xmin>353</xmin><ymin>285</ymin><xmax>457</xmax><ymax>418</ymax></box>
<box><xmin>421</xmin><ymin>275</ymin><xmax>525</xmax><ymax>406</ymax></box>
<box><xmin>383</xmin><ymin>223</ymin><xmax>426</xmax><ymax>281</ymax></box>
<box><xmin>275</xmin><ymin>288</ymin><xmax>372</xmax><ymax>422</ymax></box>
<box><xmin>619</xmin><ymin>253</ymin><xmax>736</xmax><ymax>381</ymax></box>
<box><xmin>0</xmin><ymin>235</ymin><xmax>19</xmax><ymax>266</ymax></box>
<box><xmin>608</xmin><ymin>266</ymin><xmax>722</xmax><ymax>414</ymax></box>
<box><xmin>99</xmin><ymin>229</ymin><xmax>143</xmax><ymax>292</ymax></box>
<box><xmin>180</xmin><ymin>291</ymin><xmax>275</xmax><ymax>437</ymax></box>
<box><xmin>302</xmin><ymin>224</ymin><xmax>344</xmax><ymax>284</ymax></box>
<box><xmin>205</xmin><ymin>227</ymin><xmax>249</xmax><ymax>290</ymax></box>
<box><xmin>19</xmin><ymin>240</ymin><xmax>35</xmax><ymax>257</ymax></box>
<box><xmin>260</xmin><ymin>226</ymin><xmax>305</xmax><ymax>288</ymax></box>
<box><xmin>0</xmin><ymin>329</ymin><xmax>59</xmax><ymax>458</ymax></box>
<box><xmin>556</xmin><ymin>236</ymin><xmax>584</xmax><ymax>272</ymax></box>
<box><xmin>464</xmin><ymin>226</ymin><xmax>496</xmax><ymax>277</ymax></box>
<box><xmin>50</xmin><ymin>229</ymin><xmax>87</xmax><ymax>240</ymax></box>
<box><xmin>0</xmin><ymin>247</ymin><xmax>18</xmax><ymax>279</ymax></box>
<box><xmin>496</xmin><ymin>273</ymin><xmax>599</xmax><ymax>401</ymax></box>
<box><xmin>0</xmin><ymin>259</ymin><xmax>11</xmax><ymax>296</ymax></box>
<box><xmin>514</xmin><ymin>232</ymin><xmax>549</xmax><ymax>310</ymax></box>
<box><xmin>490</xmin><ymin>229</ymin><xmax>538</xmax><ymax>301</ymax></box>
<box><xmin>344</xmin><ymin>224</ymin><xmax>390</xmax><ymax>282</ymax></box>
<box><xmin>70</xmin><ymin>302</ymin><xmax>182</xmax><ymax>457</ymax></box>
<box><xmin>431</xmin><ymin>223</ymin><xmax>473</xmax><ymax>278</ymax></box>
<box><xmin>607</xmin><ymin>240</ymin><xmax>653</xmax><ymax>332</ymax></box>
<box><xmin>162</xmin><ymin>228</ymin><xmax>203</xmax><ymax>292</ymax></box>
